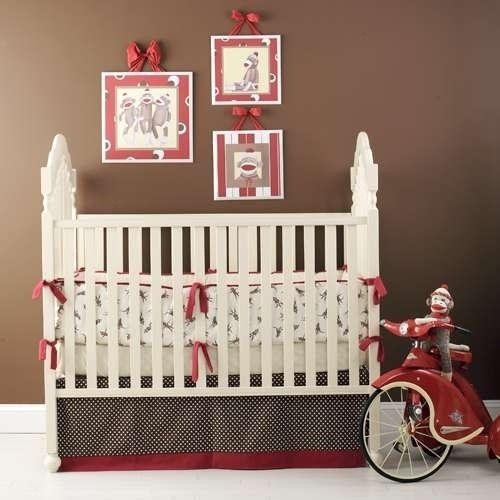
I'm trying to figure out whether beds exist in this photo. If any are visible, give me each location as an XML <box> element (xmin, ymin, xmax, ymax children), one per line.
<box><xmin>39</xmin><ymin>129</ymin><xmax>382</xmax><ymax>476</ymax></box>
